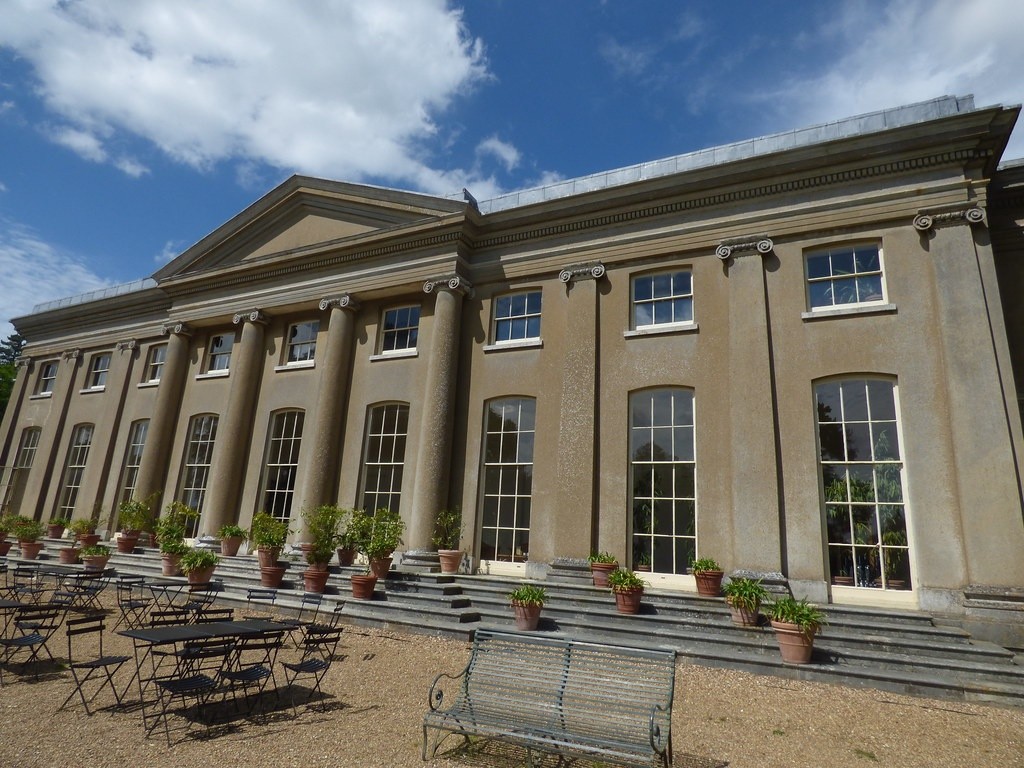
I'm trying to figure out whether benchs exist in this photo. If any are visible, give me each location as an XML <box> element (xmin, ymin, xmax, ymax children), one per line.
<box><xmin>422</xmin><ymin>625</ymin><xmax>676</xmax><ymax>768</ymax></box>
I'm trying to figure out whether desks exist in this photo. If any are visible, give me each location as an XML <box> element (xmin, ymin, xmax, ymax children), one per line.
<box><xmin>0</xmin><ymin>598</ymin><xmax>30</xmax><ymax>662</ymax></box>
<box><xmin>130</xmin><ymin>580</ymin><xmax>221</xmax><ymax>630</ymax></box>
<box><xmin>15</xmin><ymin>565</ymin><xmax>104</xmax><ymax>601</ymax></box>
<box><xmin>213</xmin><ymin>617</ymin><xmax>300</xmax><ymax>717</ymax></box>
<box><xmin>116</xmin><ymin>620</ymin><xmax>258</xmax><ymax>731</ymax></box>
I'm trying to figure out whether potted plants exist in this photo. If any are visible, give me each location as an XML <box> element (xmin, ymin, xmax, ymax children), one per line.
<box><xmin>692</xmin><ymin>557</ymin><xmax>723</xmax><ymax>598</ymax></box>
<box><xmin>0</xmin><ymin>515</ymin><xmax>44</xmax><ymax>559</ymax></box>
<box><xmin>822</xmin><ymin>252</ymin><xmax>883</xmax><ymax>301</ymax></box>
<box><xmin>724</xmin><ymin>577</ymin><xmax>770</xmax><ymax>624</ymax></box>
<box><xmin>771</xmin><ymin>594</ymin><xmax>830</xmax><ymax>665</ymax></box>
<box><xmin>433</xmin><ymin>508</ymin><xmax>467</xmax><ymax>576</ymax></box>
<box><xmin>506</xmin><ymin>584</ymin><xmax>546</xmax><ymax>631</ymax></box>
<box><xmin>47</xmin><ymin>489</ymin><xmax>406</xmax><ymax>598</ymax></box>
<box><xmin>824</xmin><ymin>429</ymin><xmax>909</xmax><ymax>589</ymax></box>
<box><xmin>587</xmin><ymin>550</ymin><xmax>651</xmax><ymax>614</ymax></box>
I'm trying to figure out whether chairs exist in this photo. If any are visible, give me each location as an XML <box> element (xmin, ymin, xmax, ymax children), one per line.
<box><xmin>0</xmin><ymin>561</ymin><xmax>345</xmax><ymax>751</ymax></box>
